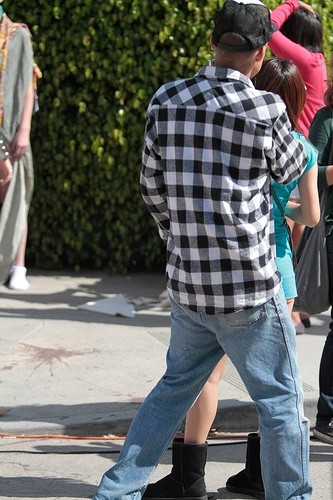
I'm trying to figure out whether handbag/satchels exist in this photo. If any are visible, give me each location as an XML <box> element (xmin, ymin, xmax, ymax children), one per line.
<box><xmin>295</xmin><ymin>186</ymin><xmax>330</xmax><ymax>315</ymax></box>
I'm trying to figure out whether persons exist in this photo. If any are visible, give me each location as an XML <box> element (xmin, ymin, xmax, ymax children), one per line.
<box><xmin>93</xmin><ymin>0</ymin><xmax>333</xmax><ymax>499</ymax></box>
<box><xmin>0</xmin><ymin>0</ymin><xmax>42</xmax><ymax>290</ymax></box>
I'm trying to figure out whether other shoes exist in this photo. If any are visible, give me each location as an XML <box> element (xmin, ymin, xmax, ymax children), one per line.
<box><xmin>295</xmin><ymin>323</ymin><xmax>306</xmax><ymax>335</ymax></box>
<box><xmin>313</xmin><ymin>426</ymin><xmax>333</xmax><ymax>443</ymax></box>
<box><xmin>9</xmin><ymin>264</ymin><xmax>30</xmax><ymax>290</ymax></box>
<box><xmin>300</xmin><ymin>317</ymin><xmax>312</xmax><ymax>328</ymax></box>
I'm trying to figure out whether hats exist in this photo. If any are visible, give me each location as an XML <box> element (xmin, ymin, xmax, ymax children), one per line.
<box><xmin>211</xmin><ymin>0</ymin><xmax>279</xmax><ymax>53</ymax></box>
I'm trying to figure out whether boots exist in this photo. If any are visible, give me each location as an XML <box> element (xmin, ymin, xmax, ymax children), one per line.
<box><xmin>143</xmin><ymin>438</ymin><xmax>208</xmax><ymax>500</ymax></box>
<box><xmin>226</xmin><ymin>432</ymin><xmax>265</xmax><ymax>499</ymax></box>
